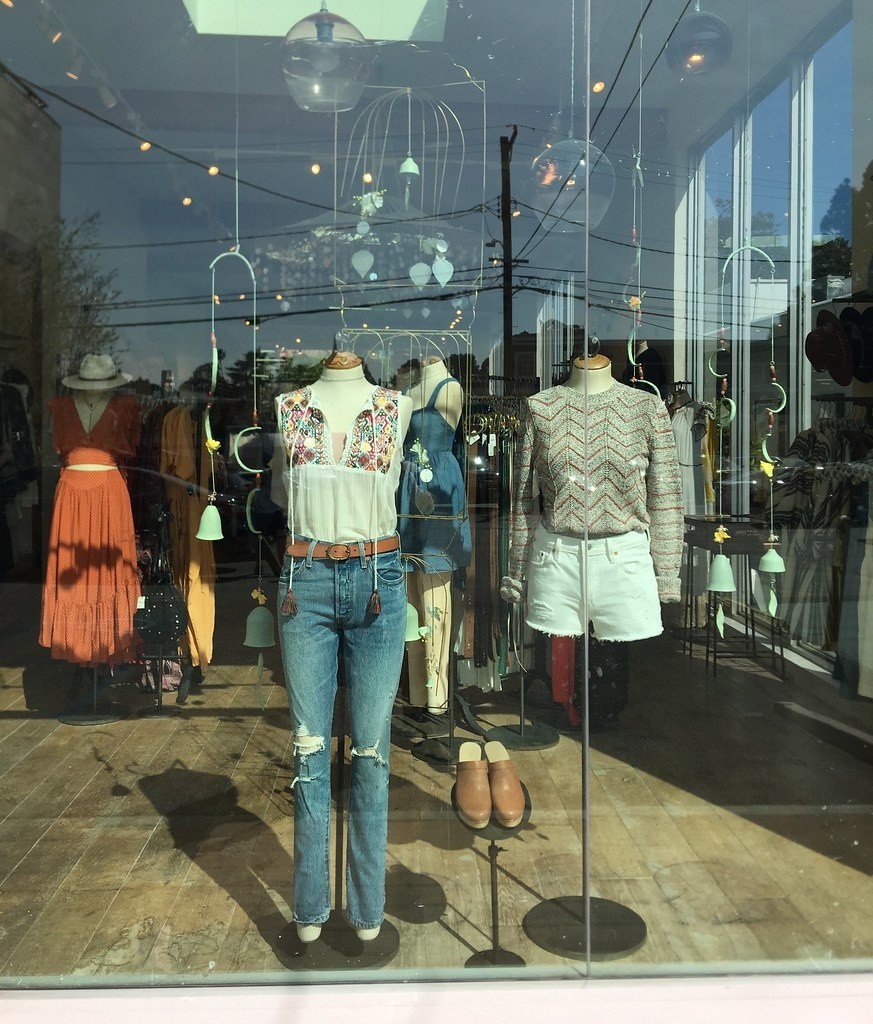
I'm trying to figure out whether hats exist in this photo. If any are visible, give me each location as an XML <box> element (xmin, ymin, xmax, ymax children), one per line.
<box><xmin>805</xmin><ymin>309</ymin><xmax>853</xmax><ymax>387</ymax></box>
<box><xmin>62</xmin><ymin>353</ymin><xmax>132</xmax><ymax>391</ymax></box>
<box><xmin>840</xmin><ymin>308</ymin><xmax>873</xmax><ymax>382</ymax></box>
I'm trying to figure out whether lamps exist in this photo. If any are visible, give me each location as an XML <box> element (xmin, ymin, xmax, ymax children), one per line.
<box><xmin>665</xmin><ymin>0</ymin><xmax>733</xmax><ymax>80</ymax></box>
<box><xmin>278</xmin><ymin>0</ymin><xmax>373</xmax><ymax>114</ymax></box>
<box><xmin>528</xmin><ymin>0</ymin><xmax>616</xmax><ymax>234</ymax></box>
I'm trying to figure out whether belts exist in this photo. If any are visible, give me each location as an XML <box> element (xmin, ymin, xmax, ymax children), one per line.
<box><xmin>284</xmin><ymin>535</ymin><xmax>401</xmax><ymax>561</ymax></box>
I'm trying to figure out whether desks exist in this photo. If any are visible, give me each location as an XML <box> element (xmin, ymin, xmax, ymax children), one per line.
<box><xmin>683</xmin><ymin>532</ymin><xmax>788</xmax><ymax>680</ymax></box>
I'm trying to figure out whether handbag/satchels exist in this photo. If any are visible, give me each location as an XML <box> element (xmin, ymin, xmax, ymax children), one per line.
<box><xmin>133</xmin><ymin>581</ymin><xmax>189</xmax><ymax>648</ymax></box>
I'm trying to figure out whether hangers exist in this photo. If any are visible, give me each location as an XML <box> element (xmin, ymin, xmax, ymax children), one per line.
<box><xmin>134</xmin><ymin>394</ymin><xmax>199</xmax><ymax>418</ymax></box>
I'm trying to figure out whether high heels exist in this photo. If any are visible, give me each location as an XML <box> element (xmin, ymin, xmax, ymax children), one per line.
<box><xmin>457</xmin><ymin>741</ymin><xmax>526</xmax><ymax>829</ymax></box>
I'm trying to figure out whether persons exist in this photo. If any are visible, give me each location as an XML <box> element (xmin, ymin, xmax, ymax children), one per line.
<box><xmin>666</xmin><ymin>389</ymin><xmax>717</xmax><ymax>520</ymax></box>
<box><xmin>510</xmin><ymin>344</ymin><xmax>686</xmax><ymax>643</ymax></box>
<box><xmin>624</xmin><ymin>325</ymin><xmax>662</xmax><ymax>394</ymax></box>
<box><xmin>396</xmin><ymin>356</ymin><xmax>477</xmax><ymax>715</ymax></box>
<box><xmin>40</xmin><ymin>354</ymin><xmax>148</xmax><ymax>668</ymax></box>
<box><xmin>270</xmin><ymin>350</ymin><xmax>414</xmax><ymax>942</ymax></box>
